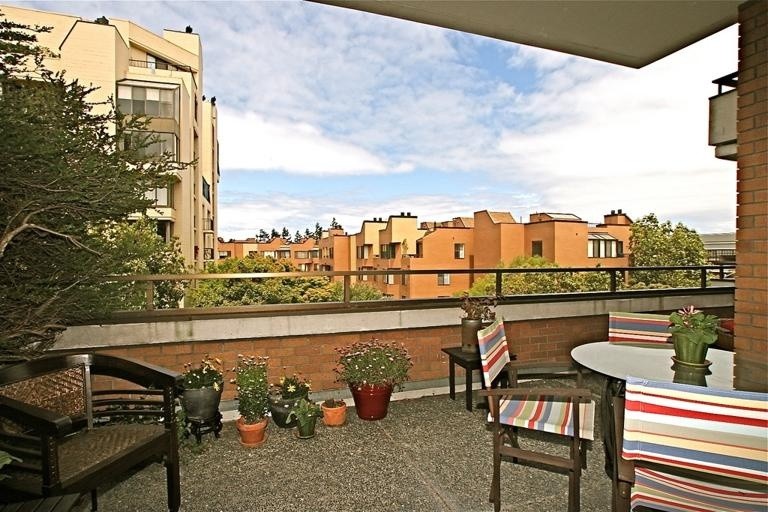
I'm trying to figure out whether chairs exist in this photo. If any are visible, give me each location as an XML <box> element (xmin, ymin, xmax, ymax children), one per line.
<box><xmin>0</xmin><ymin>349</ymin><xmax>185</xmax><ymax>511</ymax></box>
<box><xmin>606</xmin><ymin>311</ymin><xmax>676</xmax><ymax>350</ymax></box>
<box><xmin>715</xmin><ymin>317</ymin><xmax>733</xmax><ymax>352</ymax></box>
<box><xmin>608</xmin><ymin>375</ymin><xmax>767</xmax><ymax>512</ymax></box>
<box><xmin>475</xmin><ymin>315</ymin><xmax>595</xmax><ymax>512</ymax></box>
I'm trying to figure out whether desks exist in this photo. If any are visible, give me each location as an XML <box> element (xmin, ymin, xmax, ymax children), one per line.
<box><xmin>439</xmin><ymin>346</ymin><xmax>517</xmax><ymax>411</ymax></box>
<box><xmin>568</xmin><ymin>340</ymin><xmax>735</xmax><ymax>482</ymax></box>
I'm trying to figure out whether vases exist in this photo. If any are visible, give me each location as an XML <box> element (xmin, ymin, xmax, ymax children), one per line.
<box><xmin>345</xmin><ymin>380</ymin><xmax>392</xmax><ymax>422</ymax></box>
<box><xmin>669</xmin><ymin>333</ymin><xmax>712</xmax><ymax>387</ymax></box>
<box><xmin>234</xmin><ymin>417</ymin><xmax>269</xmax><ymax>447</ymax></box>
<box><xmin>269</xmin><ymin>403</ymin><xmax>299</xmax><ymax>429</ymax></box>
<box><xmin>180</xmin><ymin>380</ymin><xmax>225</xmax><ymax>421</ymax></box>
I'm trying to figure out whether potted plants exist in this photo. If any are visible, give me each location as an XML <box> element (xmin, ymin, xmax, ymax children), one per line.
<box><xmin>320</xmin><ymin>398</ymin><xmax>347</xmax><ymax>427</ymax></box>
<box><xmin>458</xmin><ymin>285</ymin><xmax>505</xmax><ymax>354</ymax></box>
<box><xmin>284</xmin><ymin>399</ymin><xmax>323</xmax><ymax>439</ymax></box>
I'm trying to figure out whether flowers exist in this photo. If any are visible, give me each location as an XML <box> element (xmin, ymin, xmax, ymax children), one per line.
<box><xmin>180</xmin><ymin>351</ymin><xmax>230</xmax><ymax>392</ymax></box>
<box><xmin>274</xmin><ymin>364</ymin><xmax>312</xmax><ymax>402</ymax></box>
<box><xmin>229</xmin><ymin>352</ymin><xmax>274</xmax><ymax>425</ymax></box>
<box><xmin>666</xmin><ymin>304</ymin><xmax>730</xmax><ymax>345</ymax></box>
<box><xmin>331</xmin><ymin>335</ymin><xmax>414</xmax><ymax>395</ymax></box>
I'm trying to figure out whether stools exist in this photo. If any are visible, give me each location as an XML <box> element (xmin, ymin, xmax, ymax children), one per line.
<box><xmin>183</xmin><ymin>413</ymin><xmax>223</xmax><ymax>443</ymax></box>
